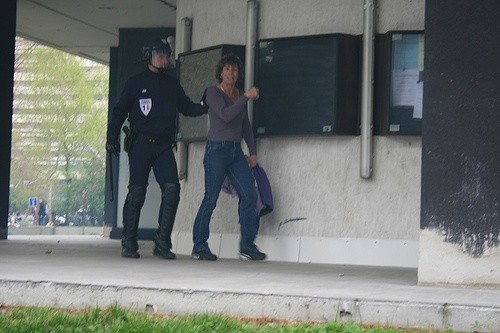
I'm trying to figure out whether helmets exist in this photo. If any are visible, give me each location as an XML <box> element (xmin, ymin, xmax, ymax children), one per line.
<box><xmin>143</xmin><ymin>37</ymin><xmax>172</xmax><ymax>59</ymax></box>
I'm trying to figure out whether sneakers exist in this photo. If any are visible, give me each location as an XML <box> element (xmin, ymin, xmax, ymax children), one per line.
<box><xmin>239</xmin><ymin>247</ymin><xmax>266</xmax><ymax>261</ymax></box>
<box><xmin>153</xmin><ymin>244</ymin><xmax>176</xmax><ymax>259</ymax></box>
<box><xmin>122</xmin><ymin>248</ymin><xmax>140</xmax><ymax>258</ymax></box>
<box><xmin>192</xmin><ymin>249</ymin><xmax>217</xmax><ymax>261</ymax></box>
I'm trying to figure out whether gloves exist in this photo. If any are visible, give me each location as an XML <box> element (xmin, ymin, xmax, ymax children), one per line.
<box><xmin>105</xmin><ymin>136</ymin><xmax>120</xmax><ymax>157</ymax></box>
<box><xmin>200</xmin><ymin>88</ymin><xmax>209</xmax><ymax>113</ymax></box>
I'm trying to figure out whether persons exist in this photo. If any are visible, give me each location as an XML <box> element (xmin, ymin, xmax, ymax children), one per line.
<box><xmin>6</xmin><ymin>197</ymin><xmax>68</xmax><ymax>228</ymax></box>
<box><xmin>105</xmin><ymin>35</ymin><xmax>210</xmax><ymax>261</ymax></box>
<box><xmin>191</xmin><ymin>55</ymin><xmax>267</xmax><ymax>262</ymax></box>
